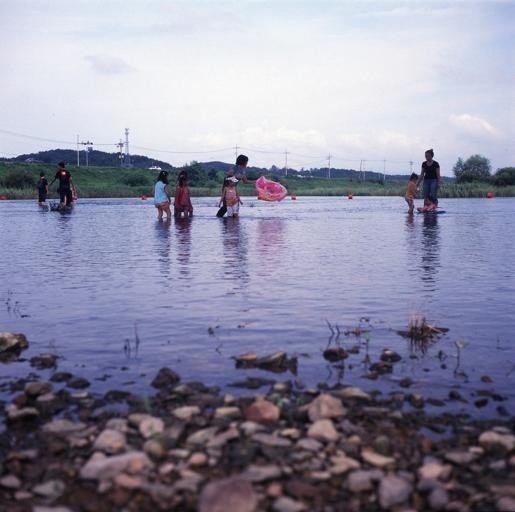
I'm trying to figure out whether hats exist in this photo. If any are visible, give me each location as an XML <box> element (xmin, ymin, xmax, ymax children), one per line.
<box><xmin>225</xmin><ymin>175</ymin><xmax>239</xmax><ymax>183</ymax></box>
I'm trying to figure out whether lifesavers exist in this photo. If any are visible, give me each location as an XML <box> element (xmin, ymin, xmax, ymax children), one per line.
<box><xmin>255</xmin><ymin>175</ymin><xmax>287</xmax><ymax>201</ymax></box>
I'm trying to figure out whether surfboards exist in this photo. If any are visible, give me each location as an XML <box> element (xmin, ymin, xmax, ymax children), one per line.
<box><xmin>417</xmin><ymin>210</ymin><xmax>446</xmax><ymax>214</ymax></box>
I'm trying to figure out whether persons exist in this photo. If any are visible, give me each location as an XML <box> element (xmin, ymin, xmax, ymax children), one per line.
<box><xmin>154</xmin><ymin>170</ymin><xmax>171</xmax><ymax>224</ymax></box>
<box><xmin>417</xmin><ymin>196</ymin><xmax>436</xmax><ymax>213</ymax></box>
<box><xmin>37</xmin><ymin>173</ymin><xmax>48</xmax><ymax>207</ymax></box>
<box><xmin>217</xmin><ymin>155</ymin><xmax>256</xmax><ymax>217</ymax></box>
<box><xmin>404</xmin><ymin>172</ymin><xmax>418</xmax><ymax>214</ymax></box>
<box><xmin>174</xmin><ymin>170</ymin><xmax>193</xmax><ymax>220</ymax></box>
<box><xmin>223</xmin><ymin>176</ymin><xmax>238</xmax><ymax>218</ymax></box>
<box><xmin>417</xmin><ymin>149</ymin><xmax>441</xmax><ymax>208</ymax></box>
<box><xmin>51</xmin><ymin>161</ymin><xmax>72</xmax><ymax>208</ymax></box>
<box><xmin>57</xmin><ymin>187</ymin><xmax>61</xmax><ymax>194</ymax></box>
